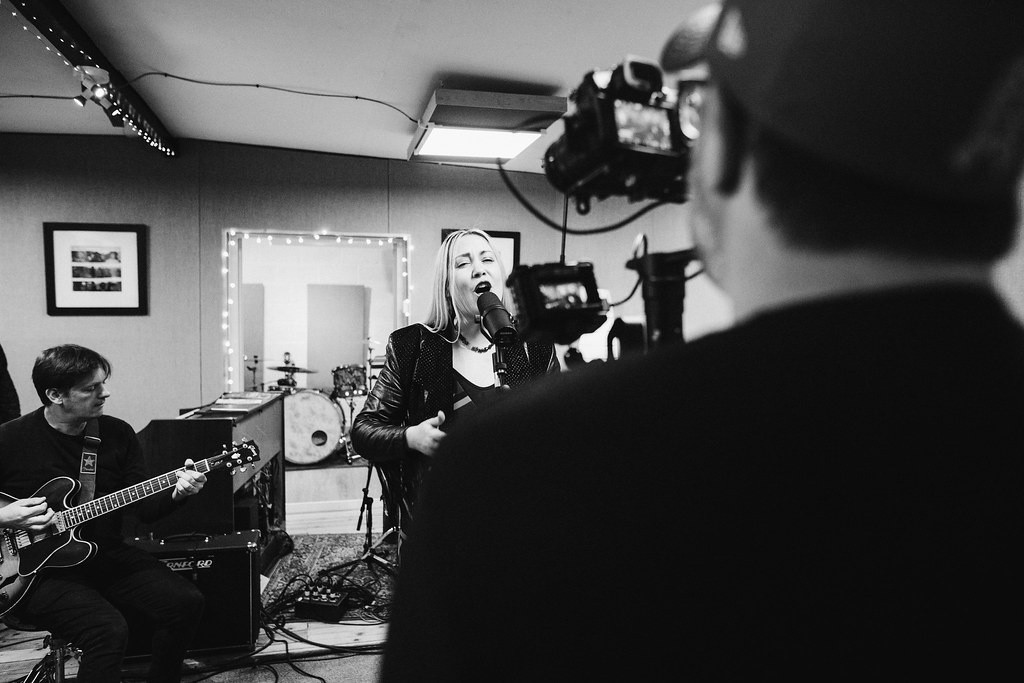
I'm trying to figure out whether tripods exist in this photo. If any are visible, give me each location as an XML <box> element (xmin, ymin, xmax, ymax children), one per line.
<box><xmin>316</xmin><ymin>461</ymin><xmax>398</xmax><ymax>584</ymax></box>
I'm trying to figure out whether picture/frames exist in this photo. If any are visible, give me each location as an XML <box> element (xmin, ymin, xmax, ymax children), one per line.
<box><xmin>43</xmin><ymin>221</ymin><xmax>148</xmax><ymax>317</ymax></box>
<box><xmin>441</xmin><ymin>228</ymin><xmax>521</xmax><ymax>279</ymax></box>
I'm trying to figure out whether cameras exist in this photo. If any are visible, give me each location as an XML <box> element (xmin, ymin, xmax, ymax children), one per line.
<box><xmin>544</xmin><ymin>56</ymin><xmax>692</xmax><ymax>205</ymax></box>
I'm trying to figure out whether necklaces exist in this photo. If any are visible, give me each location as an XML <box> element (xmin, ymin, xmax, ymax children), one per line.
<box><xmin>457</xmin><ymin>331</ymin><xmax>494</xmax><ymax>353</ymax></box>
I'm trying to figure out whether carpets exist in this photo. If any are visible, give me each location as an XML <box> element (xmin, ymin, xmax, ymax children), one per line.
<box><xmin>261</xmin><ymin>532</ymin><xmax>398</xmax><ymax>621</ymax></box>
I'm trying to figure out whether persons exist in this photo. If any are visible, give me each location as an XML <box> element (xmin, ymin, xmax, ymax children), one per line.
<box><xmin>378</xmin><ymin>0</ymin><xmax>1024</xmax><ymax>683</ymax></box>
<box><xmin>348</xmin><ymin>228</ymin><xmax>560</xmax><ymax>560</ymax></box>
<box><xmin>0</xmin><ymin>343</ymin><xmax>206</xmax><ymax>683</ymax></box>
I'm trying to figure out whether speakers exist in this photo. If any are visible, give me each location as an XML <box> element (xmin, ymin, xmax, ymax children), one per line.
<box><xmin>121</xmin><ymin>527</ymin><xmax>264</xmax><ymax>659</ymax></box>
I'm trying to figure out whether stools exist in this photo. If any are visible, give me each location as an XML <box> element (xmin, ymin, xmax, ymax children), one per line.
<box><xmin>0</xmin><ymin>609</ymin><xmax>82</xmax><ymax>683</ymax></box>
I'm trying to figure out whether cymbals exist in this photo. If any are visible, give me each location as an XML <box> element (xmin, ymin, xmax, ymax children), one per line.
<box><xmin>244</xmin><ymin>359</ymin><xmax>272</xmax><ymax>362</ymax></box>
<box><xmin>356</xmin><ymin>340</ymin><xmax>380</xmax><ymax>345</ymax></box>
<box><xmin>267</xmin><ymin>366</ymin><xmax>317</xmax><ymax>374</ymax></box>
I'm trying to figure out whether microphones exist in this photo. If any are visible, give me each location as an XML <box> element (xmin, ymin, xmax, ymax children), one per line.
<box><xmin>476</xmin><ymin>291</ymin><xmax>518</xmax><ymax>348</ymax></box>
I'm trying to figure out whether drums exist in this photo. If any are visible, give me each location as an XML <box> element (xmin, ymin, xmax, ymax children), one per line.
<box><xmin>332</xmin><ymin>363</ymin><xmax>368</xmax><ymax>398</ymax></box>
<box><xmin>281</xmin><ymin>388</ymin><xmax>345</xmax><ymax>466</ymax></box>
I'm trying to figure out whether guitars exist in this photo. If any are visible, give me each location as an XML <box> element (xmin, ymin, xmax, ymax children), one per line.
<box><xmin>0</xmin><ymin>435</ymin><xmax>261</xmax><ymax>613</ymax></box>
